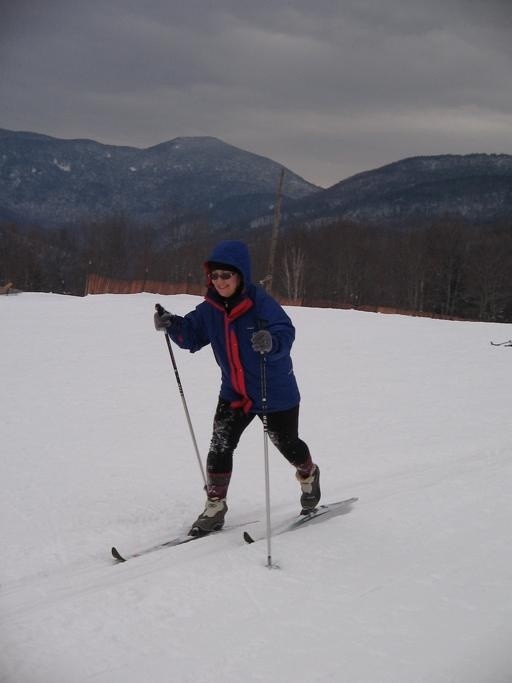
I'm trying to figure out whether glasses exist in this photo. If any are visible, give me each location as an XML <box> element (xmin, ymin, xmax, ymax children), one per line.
<box><xmin>210</xmin><ymin>271</ymin><xmax>237</xmax><ymax>279</ymax></box>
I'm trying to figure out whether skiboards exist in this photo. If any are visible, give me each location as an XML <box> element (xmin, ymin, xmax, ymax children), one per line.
<box><xmin>112</xmin><ymin>499</ymin><xmax>359</xmax><ymax>563</ymax></box>
<box><xmin>491</xmin><ymin>341</ymin><xmax>512</xmax><ymax>347</ymax></box>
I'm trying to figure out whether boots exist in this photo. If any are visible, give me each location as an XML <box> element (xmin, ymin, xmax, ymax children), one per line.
<box><xmin>296</xmin><ymin>464</ymin><xmax>321</xmax><ymax>509</ymax></box>
<box><xmin>187</xmin><ymin>498</ymin><xmax>228</xmax><ymax>537</ymax></box>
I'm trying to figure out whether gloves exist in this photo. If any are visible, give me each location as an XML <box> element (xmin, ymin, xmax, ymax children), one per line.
<box><xmin>154</xmin><ymin>310</ymin><xmax>172</xmax><ymax>331</ymax></box>
<box><xmin>250</xmin><ymin>330</ymin><xmax>273</xmax><ymax>353</ymax></box>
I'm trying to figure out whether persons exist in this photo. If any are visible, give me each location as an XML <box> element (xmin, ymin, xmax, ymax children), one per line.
<box><xmin>154</xmin><ymin>240</ymin><xmax>321</xmax><ymax>533</ymax></box>
